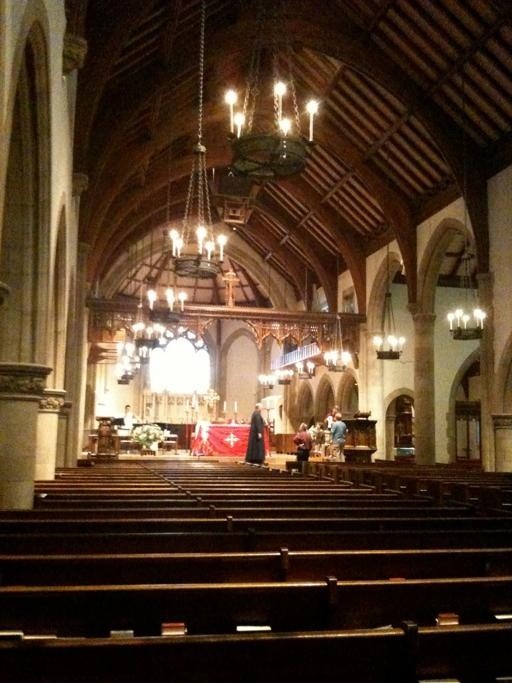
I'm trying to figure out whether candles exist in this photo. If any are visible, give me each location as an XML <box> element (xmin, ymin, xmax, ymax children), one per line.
<box><xmin>185</xmin><ymin>393</ymin><xmax>239</xmax><ymax>416</ymax></box>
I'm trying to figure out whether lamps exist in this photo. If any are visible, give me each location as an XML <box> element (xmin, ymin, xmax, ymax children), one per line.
<box><xmin>372</xmin><ymin>223</ymin><xmax>407</xmax><ymax>360</ymax></box>
<box><xmin>168</xmin><ymin>2</ymin><xmax>229</xmax><ymax>278</ymax></box>
<box><xmin>448</xmin><ymin>190</ymin><xmax>488</xmax><ymax>340</ymax></box>
<box><xmin>259</xmin><ymin>186</ymin><xmax>353</xmax><ymax>386</ymax></box>
<box><xmin>223</xmin><ymin>1</ymin><xmax>320</xmax><ymax>179</ymax></box>
<box><xmin>116</xmin><ymin>289</ymin><xmax>187</xmax><ymax>386</ymax></box>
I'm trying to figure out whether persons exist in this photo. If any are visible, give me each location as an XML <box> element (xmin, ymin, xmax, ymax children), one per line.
<box><xmin>293</xmin><ymin>409</ymin><xmax>349</xmax><ymax>473</ymax></box>
<box><xmin>245</xmin><ymin>404</ymin><xmax>264</xmax><ymax>464</ymax></box>
<box><xmin>122</xmin><ymin>405</ymin><xmax>133</xmax><ymax>454</ymax></box>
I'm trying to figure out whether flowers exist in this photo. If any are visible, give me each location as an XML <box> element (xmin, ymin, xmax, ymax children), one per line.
<box><xmin>133</xmin><ymin>423</ymin><xmax>164</xmax><ymax>446</ymax></box>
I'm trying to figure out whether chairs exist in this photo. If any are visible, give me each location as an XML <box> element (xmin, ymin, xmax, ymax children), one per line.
<box><xmin>0</xmin><ymin>462</ymin><xmax>510</xmax><ymax>683</ymax></box>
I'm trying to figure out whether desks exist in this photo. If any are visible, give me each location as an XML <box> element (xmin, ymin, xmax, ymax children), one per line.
<box><xmin>87</xmin><ymin>432</ymin><xmax>179</xmax><ymax>455</ymax></box>
<box><xmin>189</xmin><ymin>423</ymin><xmax>270</xmax><ymax>457</ymax></box>
